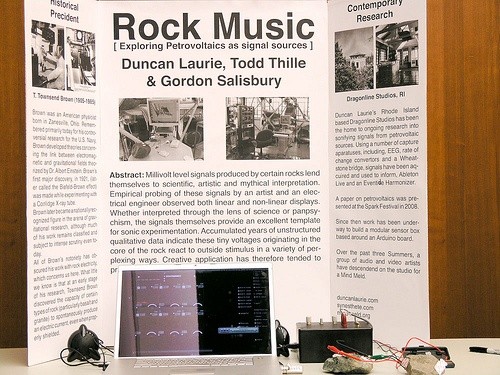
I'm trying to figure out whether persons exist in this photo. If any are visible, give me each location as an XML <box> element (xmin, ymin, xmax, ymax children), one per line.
<box><xmin>39</xmin><ymin>46</ymin><xmax>65</xmax><ymax>91</ymax></box>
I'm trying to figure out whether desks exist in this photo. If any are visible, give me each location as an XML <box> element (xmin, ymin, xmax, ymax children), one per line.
<box><xmin>0</xmin><ymin>337</ymin><xmax>500</xmax><ymax>374</ymax></box>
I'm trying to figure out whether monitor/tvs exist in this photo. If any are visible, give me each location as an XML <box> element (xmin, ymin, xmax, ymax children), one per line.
<box><xmin>147</xmin><ymin>98</ymin><xmax>181</xmax><ymax>127</ymax></box>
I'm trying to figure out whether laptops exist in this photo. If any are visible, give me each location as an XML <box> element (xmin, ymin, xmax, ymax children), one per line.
<box><xmin>101</xmin><ymin>262</ymin><xmax>281</xmax><ymax>375</ymax></box>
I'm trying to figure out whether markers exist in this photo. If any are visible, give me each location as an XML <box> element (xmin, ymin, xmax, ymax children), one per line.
<box><xmin>468</xmin><ymin>345</ymin><xmax>500</xmax><ymax>355</ymax></box>
